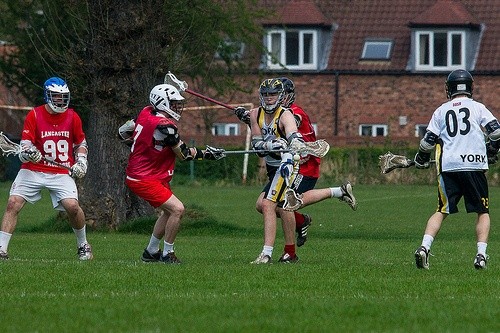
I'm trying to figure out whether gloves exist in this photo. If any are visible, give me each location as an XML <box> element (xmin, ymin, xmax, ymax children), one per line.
<box><xmin>414</xmin><ymin>154</ymin><xmax>429</xmax><ymax>169</ymax></box>
<box><xmin>205</xmin><ymin>145</ymin><xmax>225</xmax><ymax>160</ymax></box>
<box><xmin>69</xmin><ymin>161</ymin><xmax>87</xmax><ymax>177</ymax></box>
<box><xmin>24</xmin><ymin>148</ymin><xmax>42</xmax><ymax>162</ymax></box>
<box><xmin>281</xmin><ymin>162</ymin><xmax>293</xmax><ymax>177</ymax></box>
<box><xmin>234</xmin><ymin>107</ymin><xmax>251</xmax><ymax>124</ymax></box>
<box><xmin>487</xmin><ymin>150</ymin><xmax>498</xmax><ymax>164</ymax></box>
<box><xmin>269</xmin><ymin>138</ymin><xmax>287</xmax><ymax>156</ymax></box>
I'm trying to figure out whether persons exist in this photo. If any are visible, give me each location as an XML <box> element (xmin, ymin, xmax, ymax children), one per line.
<box><xmin>236</xmin><ymin>77</ymin><xmax>358</xmax><ymax>247</ymax></box>
<box><xmin>250</xmin><ymin>78</ymin><xmax>310</xmax><ymax>263</ymax></box>
<box><xmin>414</xmin><ymin>70</ymin><xmax>500</xmax><ymax>269</ymax></box>
<box><xmin>125</xmin><ymin>85</ymin><xmax>203</xmax><ymax>262</ymax></box>
<box><xmin>0</xmin><ymin>77</ymin><xmax>93</xmax><ymax>259</ymax></box>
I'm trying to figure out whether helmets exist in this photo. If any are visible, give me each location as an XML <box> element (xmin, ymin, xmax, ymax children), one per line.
<box><xmin>44</xmin><ymin>77</ymin><xmax>70</xmax><ymax>112</ymax></box>
<box><xmin>278</xmin><ymin>77</ymin><xmax>295</xmax><ymax>109</ymax></box>
<box><xmin>445</xmin><ymin>70</ymin><xmax>474</xmax><ymax>99</ymax></box>
<box><xmin>260</xmin><ymin>79</ymin><xmax>285</xmax><ymax>114</ymax></box>
<box><xmin>150</xmin><ymin>84</ymin><xmax>185</xmax><ymax>121</ymax></box>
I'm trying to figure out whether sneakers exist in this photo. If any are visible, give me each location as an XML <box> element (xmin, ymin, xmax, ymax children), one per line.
<box><xmin>142</xmin><ymin>247</ymin><xmax>162</xmax><ymax>262</ymax></box>
<box><xmin>77</xmin><ymin>243</ymin><xmax>93</xmax><ymax>260</ymax></box>
<box><xmin>296</xmin><ymin>214</ymin><xmax>311</xmax><ymax>247</ymax></box>
<box><xmin>415</xmin><ymin>246</ymin><xmax>430</xmax><ymax>269</ymax></box>
<box><xmin>0</xmin><ymin>246</ymin><xmax>8</xmax><ymax>259</ymax></box>
<box><xmin>159</xmin><ymin>250</ymin><xmax>180</xmax><ymax>263</ymax></box>
<box><xmin>473</xmin><ymin>253</ymin><xmax>486</xmax><ymax>270</ymax></box>
<box><xmin>338</xmin><ymin>183</ymin><xmax>357</xmax><ymax>211</ymax></box>
<box><xmin>278</xmin><ymin>254</ymin><xmax>298</xmax><ymax>264</ymax></box>
<box><xmin>250</xmin><ymin>253</ymin><xmax>270</xmax><ymax>264</ymax></box>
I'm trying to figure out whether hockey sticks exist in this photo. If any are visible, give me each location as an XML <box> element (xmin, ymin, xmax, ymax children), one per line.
<box><xmin>221</xmin><ymin>137</ymin><xmax>331</xmax><ymax>158</ymax></box>
<box><xmin>278</xmin><ymin>147</ymin><xmax>305</xmax><ymax>212</ymax></box>
<box><xmin>163</xmin><ymin>72</ymin><xmax>237</xmax><ymax>112</ymax></box>
<box><xmin>377</xmin><ymin>152</ymin><xmax>436</xmax><ymax>174</ymax></box>
<box><xmin>0</xmin><ymin>131</ymin><xmax>72</xmax><ymax>171</ymax></box>
